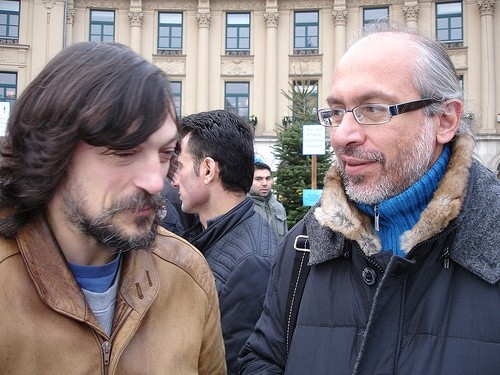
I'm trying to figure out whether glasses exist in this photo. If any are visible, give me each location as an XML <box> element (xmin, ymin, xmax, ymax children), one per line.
<box><xmin>317</xmin><ymin>98</ymin><xmax>438</xmax><ymax>127</ymax></box>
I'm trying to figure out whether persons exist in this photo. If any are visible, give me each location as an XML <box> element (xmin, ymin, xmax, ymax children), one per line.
<box><xmin>247</xmin><ymin>162</ymin><xmax>288</xmax><ymax>244</ymax></box>
<box><xmin>0</xmin><ymin>41</ymin><xmax>227</xmax><ymax>375</ymax></box>
<box><xmin>236</xmin><ymin>31</ymin><xmax>500</xmax><ymax>375</ymax></box>
<box><xmin>156</xmin><ymin>109</ymin><xmax>279</xmax><ymax>375</ymax></box>
